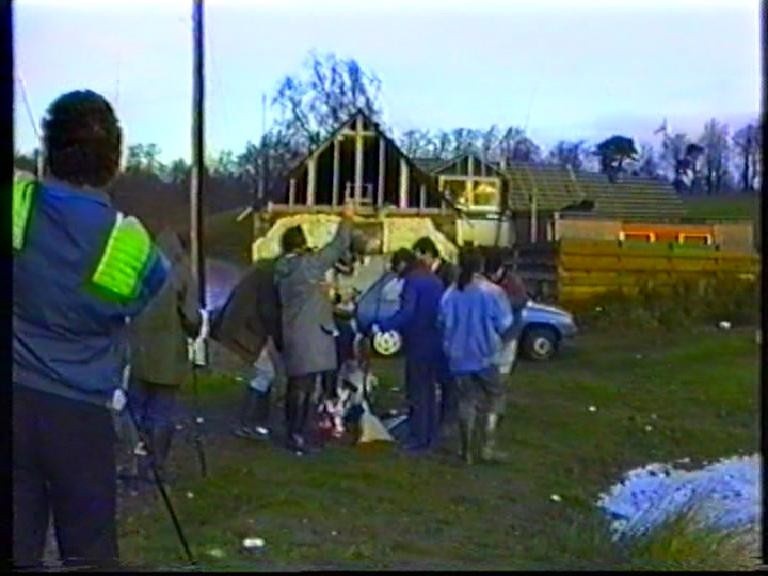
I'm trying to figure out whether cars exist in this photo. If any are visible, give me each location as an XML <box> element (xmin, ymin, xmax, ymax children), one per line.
<box><xmin>355</xmin><ymin>271</ymin><xmax>578</xmax><ymax>361</ymax></box>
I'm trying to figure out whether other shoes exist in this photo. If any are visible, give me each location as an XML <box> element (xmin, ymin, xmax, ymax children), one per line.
<box><xmin>287</xmin><ymin>432</ymin><xmax>322</xmax><ymax>455</ymax></box>
<box><xmin>238</xmin><ymin>423</ymin><xmax>273</xmax><ymax>443</ymax></box>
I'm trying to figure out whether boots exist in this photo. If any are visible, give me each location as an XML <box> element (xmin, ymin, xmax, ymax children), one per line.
<box><xmin>458</xmin><ymin>430</ymin><xmax>473</xmax><ymax>466</ymax></box>
<box><xmin>480</xmin><ymin>412</ymin><xmax>510</xmax><ymax>465</ymax></box>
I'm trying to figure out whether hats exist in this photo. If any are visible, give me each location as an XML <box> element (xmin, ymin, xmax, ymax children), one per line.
<box><xmin>484</xmin><ymin>253</ymin><xmax>501</xmax><ymax>273</ymax></box>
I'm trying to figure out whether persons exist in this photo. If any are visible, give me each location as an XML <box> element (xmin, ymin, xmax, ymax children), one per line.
<box><xmin>126</xmin><ymin>207</ymin><xmax>527</xmax><ymax>494</ymax></box>
<box><xmin>10</xmin><ymin>89</ymin><xmax>171</xmax><ymax>568</ymax></box>
